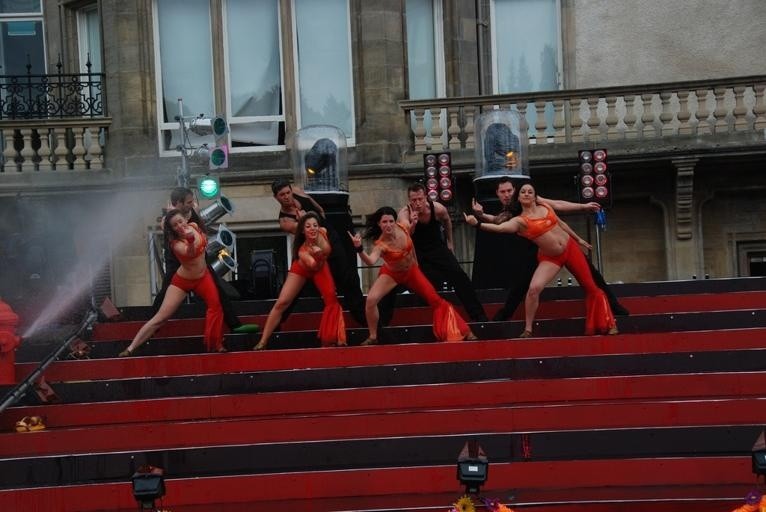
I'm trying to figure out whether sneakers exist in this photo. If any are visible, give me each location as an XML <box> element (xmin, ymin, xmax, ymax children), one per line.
<box><xmin>464</xmin><ymin>335</ymin><xmax>479</xmax><ymax>340</ymax></box>
<box><xmin>517</xmin><ymin>329</ymin><xmax>535</xmax><ymax>338</ymax></box>
<box><xmin>118</xmin><ymin>347</ymin><xmax>132</xmax><ymax>357</ymax></box>
<box><xmin>253</xmin><ymin>340</ymin><xmax>273</xmax><ymax>350</ymax></box>
<box><xmin>360</xmin><ymin>337</ymin><xmax>378</xmax><ymax>346</ymax></box>
<box><xmin>203</xmin><ymin>338</ymin><xmax>228</xmax><ymax>353</ymax></box>
<box><xmin>608</xmin><ymin>327</ymin><xmax>620</xmax><ymax>334</ymax></box>
<box><xmin>333</xmin><ymin>339</ymin><xmax>348</xmax><ymax>346</ymax></box>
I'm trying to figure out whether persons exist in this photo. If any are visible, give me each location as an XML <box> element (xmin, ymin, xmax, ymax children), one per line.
<box><xmin>251</xmin><ymin>213</ymin><xmax>351</xmax><ymax>351</ymax></box>
<box><xmin>470</xmin><ymin>178</ymin><xmax>635</xmax><ymax>322</ymax></box>
<box><xmin>116</xmin><ymin>207</ymin><xmax>230</xmax><ymax>357</ymax></box>
<box><xmin>376</xmin><ymin>183</ymin><xmax>488</xmax><ymax>329</ymax></box>
<box><xmin>0</xmin><ymin>296</ymin><xmax>25</xmax><ymax>434</ymax></box>
<box><xmin>269</xmin><ymin>176</ymin><xmax>371</xmax><ymax>332</ymax></box>
<box><xmin>143</xmin><ymin>186</ymin><xmax>247</xmax><ymax>337</ymax></box>
<box><xmin>460</xmin><ymin>180</ymin><xmax>619</xmax><ymax>339</ymax></box>
<box><xmin>345</xmin><ymin>204</ymin><xmax>480</xmax><ymax>347</ymax></box>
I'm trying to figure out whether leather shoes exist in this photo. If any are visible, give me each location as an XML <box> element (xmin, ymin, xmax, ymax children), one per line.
<box><xmin>476</xmin><ymin>313</ymin><xmax>488</xmax><ymax>323</ymax></box>
<box><xmin>358</xmin><ymin>316</ymin><xmax>389</xmax><ymax>328</ymax></box>
<box><xmin>493</xmin><ymin>310</ymin><xmax>508</xmax><ymax>321</ymax></box>
<box><xmin>613</xmin><ymin>304</ymin><xmax>629</xmax><ymax>316</ymax></box>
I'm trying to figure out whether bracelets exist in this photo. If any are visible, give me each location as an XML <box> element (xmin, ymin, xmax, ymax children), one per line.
<box><xmin>474</xmin><ymin>221</ymin><xmax>482</xmax><ymax>229</ymax></box>
<box><xmin>351</xmin><ymin>245</ymin><xmax>364</xmax><ymax>254</ymax></box>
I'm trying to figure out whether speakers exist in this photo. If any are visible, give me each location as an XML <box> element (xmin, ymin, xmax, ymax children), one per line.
<box><xmin>305</xmin><ymin>192</ymin><xmax>357</xmax><ymax>295</ymax></box>
<box><xmin>473</xmin><ymin>175</ymin><xmax>533</xmax><ymax>289</ymax></box>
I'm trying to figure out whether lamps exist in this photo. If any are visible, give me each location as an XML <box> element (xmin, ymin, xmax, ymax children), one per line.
<box><xmin>575</xmin><ymin>148</ymin><xmax>621</xmax><ymax>288</ymax></box>
<box><xmin>422</xmin><ymin>150</ymin><xmax>457</xmax><ymax>234</ymax></box>
<box><xmin>174</xmin><ymin>98</ymin><xmax>238</xmax><ymax>279</ymax></box>
<box><xmin>456</xmin><ymin>440</ymin><xmax>489</xmax><ymax>492</ymax></box>
<box><xmin>131</xmin><ymin>462</ymin><xmax>166</xmax><ymax>512</ymax></box>
<box><xmin>752</xmin><ymin>429</ymin><xmax>766</xmax><ymax>480</ymax></box>
